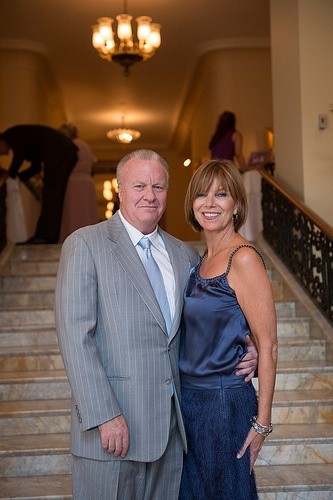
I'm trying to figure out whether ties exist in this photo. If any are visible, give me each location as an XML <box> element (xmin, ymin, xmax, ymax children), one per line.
<box><xmin>138</xmin><ymin>237</ymin><xmax>173</xmax><ymax>398</ymax></box>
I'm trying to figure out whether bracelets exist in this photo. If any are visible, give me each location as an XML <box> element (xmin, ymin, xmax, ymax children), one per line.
<box><xmin>250</xmin><ymin>414</ymin><xmax>274</xmax><ymax>437</ymax></box>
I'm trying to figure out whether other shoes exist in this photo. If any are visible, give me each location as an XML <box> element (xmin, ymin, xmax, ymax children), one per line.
<box><xmin>15</xmin><ymin>235</ymin><xmax>58</xmax><ymax>246</ymax></box>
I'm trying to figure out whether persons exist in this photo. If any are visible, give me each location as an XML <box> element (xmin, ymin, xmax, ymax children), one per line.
<box><xmin>0</xmin><ymin>124</ymin><xmax>80</xmax><ymax>244</ymax></box>
<box><xmin>209</xmin><ymin>110</ymin><xmax>246</xmax><ymax>174</ymax></box>
<box><xmin>250</xmin><ymin>128</ymin><xmax>276</xmax><ymax>174</ymax></box>
<box><xmin>56</xmin><ymin>123</ymin><xmax>97</xmax><ymax>244</ymax></box>
<box><xmin>178</xmin><ymin>158</ymin><xmax>279</xmax><ymax>500</ymax></box>
<box><xmin>54</xmin><ymin>149</ymin><xmax>259</xmax><ymax>500</ymax></box>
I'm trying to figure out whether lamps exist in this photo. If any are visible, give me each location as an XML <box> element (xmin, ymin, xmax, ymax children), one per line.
<box><xmin>105</xmin><ymin>121</ymin><xmax>140</xmax><ymax>144</ymax></box>
<box><xmin>90</xmin><ymin>0</ymin><xmax>161</xmax><ymax>78</ymax></box>
